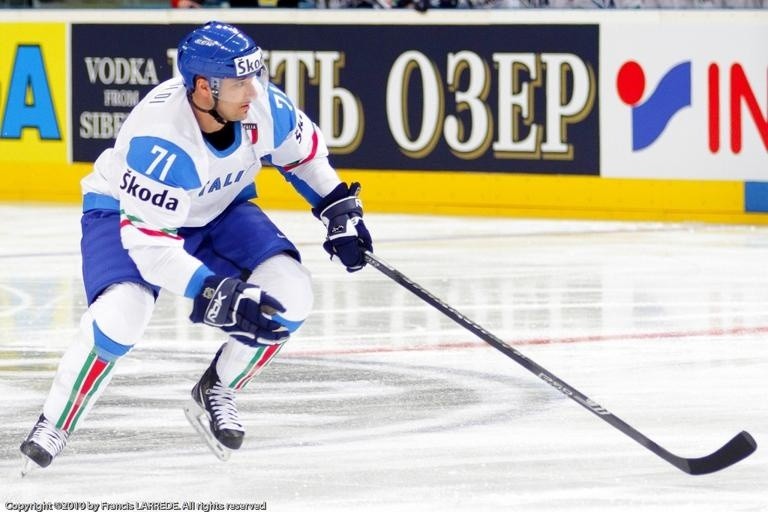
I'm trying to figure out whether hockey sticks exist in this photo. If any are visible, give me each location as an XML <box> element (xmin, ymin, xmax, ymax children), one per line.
<box><xmin>364</xmin><ymin>249</ymin><xmax>756</xmax><ymax>475</ymax></box>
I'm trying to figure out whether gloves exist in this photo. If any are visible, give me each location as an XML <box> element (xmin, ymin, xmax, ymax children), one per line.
<box><xmin>189</xmin><ymin>275</ymin><xmax>293</xmax><ymax>349</ymax></box>
<box><xmin>310</xmin><ymin>181</ymin><xmax>375</xmax><ymax>274</ymax></box>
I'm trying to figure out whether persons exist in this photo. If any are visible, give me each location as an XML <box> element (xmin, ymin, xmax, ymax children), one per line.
<box><xmin>20</xmin><ymin>22</ymin><xmax>375</xmax><ymax>479</ymax></box>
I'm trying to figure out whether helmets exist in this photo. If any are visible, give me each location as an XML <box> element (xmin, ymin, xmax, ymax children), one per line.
<box><xmin>176</xmin><ymin>17</ymin><xmax>271</xmax><ymax>104</ymax></box>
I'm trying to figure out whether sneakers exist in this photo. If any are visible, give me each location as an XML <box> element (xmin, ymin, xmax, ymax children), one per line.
<box><xmin>191</xmin><ymin>367</ymin><xmax>247</xmax><ymax>449</ymax></box>
<box><xmin>20</xmin><ymin>413</ymin><xmax>72</xmax><ymax>469</ymax></box>
